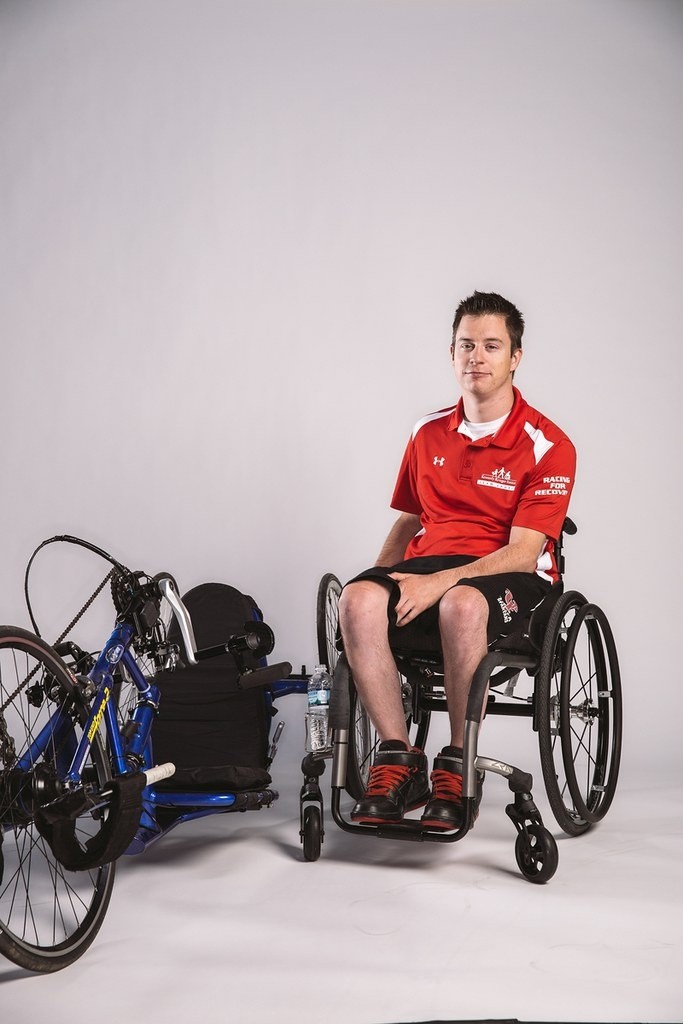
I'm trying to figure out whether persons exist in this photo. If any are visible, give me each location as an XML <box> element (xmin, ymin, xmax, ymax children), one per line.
<box><xmin>339</xmin><ymin>290</ymin><xmax>576</xmax><ymax>832</ymax></box>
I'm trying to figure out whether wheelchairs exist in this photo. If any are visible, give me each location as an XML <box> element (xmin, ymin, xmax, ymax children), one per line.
<box><xmin>299</xmin><ymin>505</ymin><xmax>623</xmax><ymax>886</ymax></box>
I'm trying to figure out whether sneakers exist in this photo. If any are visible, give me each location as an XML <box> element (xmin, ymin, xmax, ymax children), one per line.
<box><xmin>349</xmin><ymin>739</ymin><xmax>432</xmax><ymax>822</ymax></box>
<box><xmin>418</xmin><ymin>745</ymin><xmax>485</xmax><ymax>830</ymax></box>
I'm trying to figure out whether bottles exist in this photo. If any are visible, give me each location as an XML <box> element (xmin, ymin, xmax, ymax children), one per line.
<box><xmin>307</xmin><ymin>663</ymin><xmax>333</xmax><ymax>750</ymax></box>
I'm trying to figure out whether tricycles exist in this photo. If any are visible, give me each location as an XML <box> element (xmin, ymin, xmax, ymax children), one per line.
<box><xmin>0</xmin><ymin>532</ymin><xmax>295</xmax><ymax>973</ymax></box>
